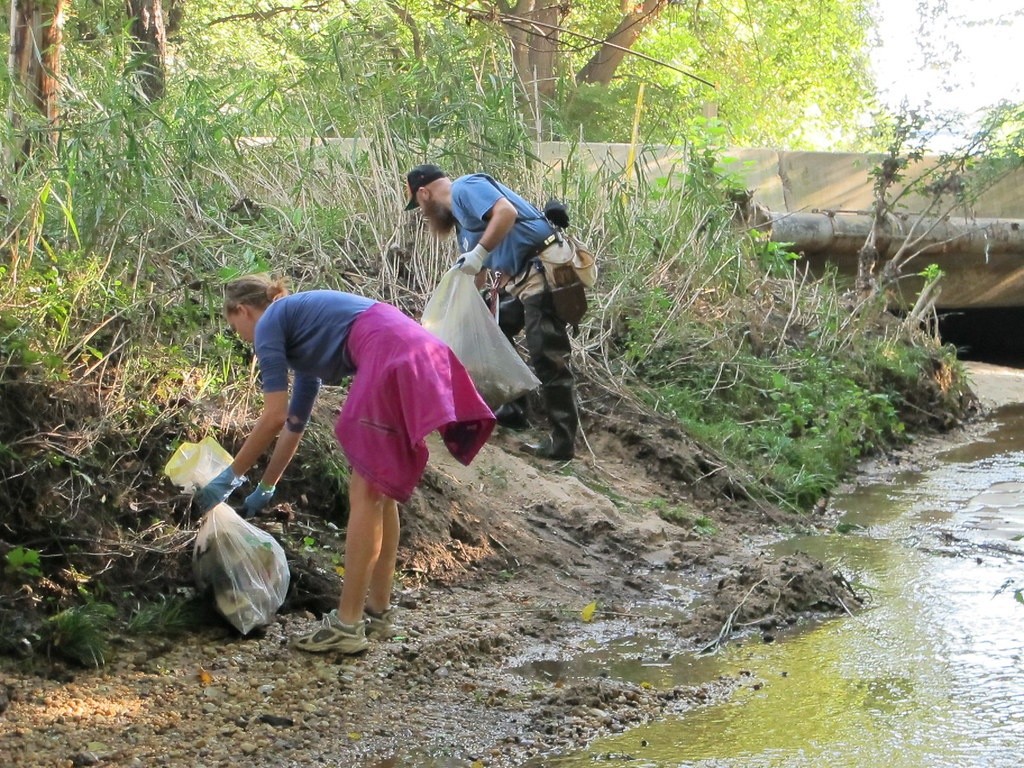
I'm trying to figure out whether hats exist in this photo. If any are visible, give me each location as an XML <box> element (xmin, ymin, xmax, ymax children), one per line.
<box><xmin>403</xmin><ymin>165</ymin><xmax>443</xmax><ymax>210</ymax></box>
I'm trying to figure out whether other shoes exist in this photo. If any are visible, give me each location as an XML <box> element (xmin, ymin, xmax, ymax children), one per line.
<box><xmin>493</xmin><ymin>406</ymin><xmax>529</xmax><ymax>430</ymax></box>
<box><xmin>519</xmin><ymin>440</ymin><xmax>575</xmax><ymax>460</ymax></box>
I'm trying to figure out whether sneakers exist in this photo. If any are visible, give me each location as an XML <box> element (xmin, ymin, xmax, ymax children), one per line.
<box><xmin>290</xmin><ymin>609</ymin><xmax>368</xmax><ymax>655</ymax></box>
<box><xmin>330</xmin><ymin>604</ymin><xmax>397</xmax><ymax>639</ymax></box>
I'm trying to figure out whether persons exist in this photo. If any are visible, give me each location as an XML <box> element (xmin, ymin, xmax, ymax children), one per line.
<box><xmin>402</xmin><ymin>163</ymin><xmax>586</xmax><ymax>461</ymax></box>
<box><xmin>166</xmin><ymin>273</ymin><xmax>451</xmax><ymax>657</ymax></box>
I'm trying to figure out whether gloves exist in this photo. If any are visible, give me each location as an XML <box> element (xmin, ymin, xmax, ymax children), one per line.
<box><xmin>242</xmin><ymin>480</ymin><xmax>276</xmax><ymax>519</ymax></box>
<box><xmin>193</xmin><ymin>466</ymin><xmax>247</xmax><ymax>513</ymax></box>
<box><xmin>455</xmin><ymin>244</ymin><xmax>488</xmax><ymax>275</ymax></box>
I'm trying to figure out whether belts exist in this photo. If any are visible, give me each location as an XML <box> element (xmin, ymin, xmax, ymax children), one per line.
<box><xmin>521</xmin><ymin>231</ymin><xmax>557</xmax><ymax>268</ymax></box>
